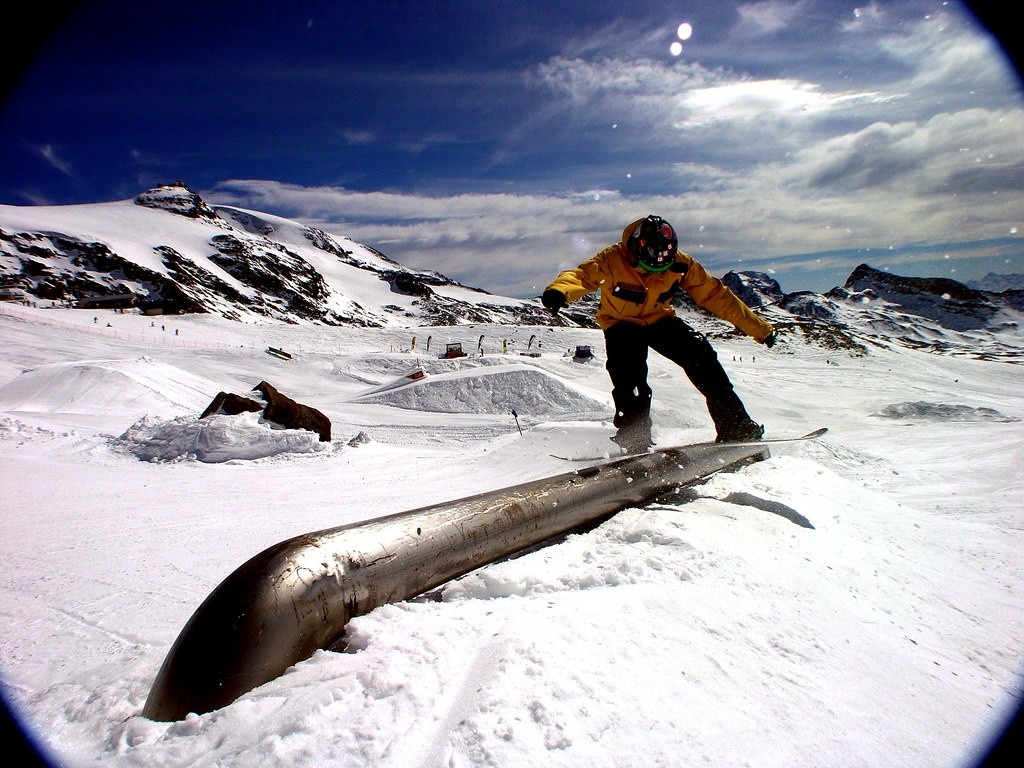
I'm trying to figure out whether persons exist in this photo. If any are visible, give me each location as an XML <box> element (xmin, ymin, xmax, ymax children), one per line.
<box><xmin>732</xmin><ymin>355</ymin><xmax>756</xmax><ymax>363</ymax></box>
<box><xmin>93</xmin><ymin>308</ymin><xmax>124</xmax><ymax>327</ymax></box>
<box><xmin>541</xmin><ymin>214</ymin><xmax>777</xmax><ymax>453</ymax></box>
<box><xmin>152</xmin><ymin>321</ymin><xmax>179</xmax><ymax>335</ymax></box>
<box><xmin>22</xmin><ymin>297</ymin><xmax>73</xmax><ymax>309</ymax></box>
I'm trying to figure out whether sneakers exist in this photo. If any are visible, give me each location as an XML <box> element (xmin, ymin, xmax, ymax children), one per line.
<box><xmin>718</xmin><ymin>419</ymin><xmax>765</xmax><ymax>443</ymax></box>
<box><xmin>617</xmin><ymin>416</ymin><xmax>656</xmax><ymax>455</ymax></box>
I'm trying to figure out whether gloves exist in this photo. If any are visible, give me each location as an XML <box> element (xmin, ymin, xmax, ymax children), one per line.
<box><xmin>542</xmin><ymin>289</ymin><xmax>569</xmax><ymax>316</ymax></box>
<box><xmin>764</xmin><ymin>331</ymin><xmax>776</xmax><ymax>348</ymax></box>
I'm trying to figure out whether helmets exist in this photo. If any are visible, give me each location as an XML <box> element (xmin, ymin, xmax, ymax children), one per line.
<box><xmin>632</xmin><ymin>216</ymin><xmax>678</xmax><ymax>274</ymax></box>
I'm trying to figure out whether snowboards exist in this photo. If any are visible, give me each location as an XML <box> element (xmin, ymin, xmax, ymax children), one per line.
<box><xmin>548</xmin><ymin>426</ymin><xmax>829</xmax><ymax>464</ymax></box>
